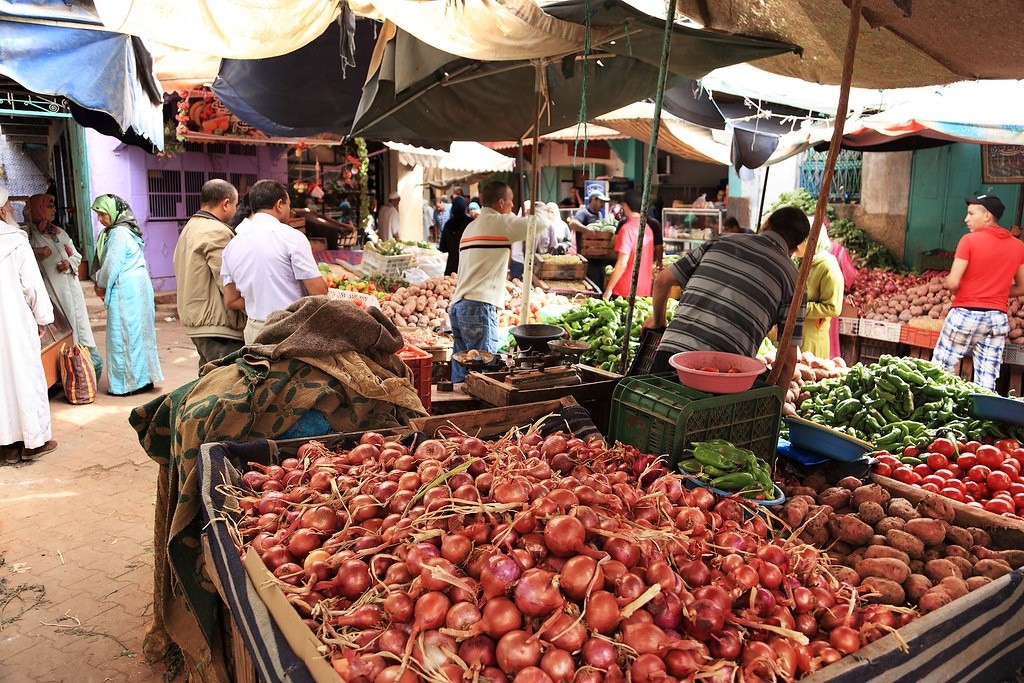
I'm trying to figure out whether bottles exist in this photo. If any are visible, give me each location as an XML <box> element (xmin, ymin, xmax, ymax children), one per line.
<box><xmin>664</xmin><ymin>222</ymin><xmax>675</xmax><ymax>237</ymax></box>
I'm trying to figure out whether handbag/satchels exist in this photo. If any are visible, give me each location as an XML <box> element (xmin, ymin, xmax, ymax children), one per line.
<box><xmin>59</xmin><ymin>341</ymin><xmax>98</xmax><ymax>405</ymax></box>
<box><xmin>90</xmin><ymin>349</ymin><xmax>103</xmax><ymax>383</ymax></box>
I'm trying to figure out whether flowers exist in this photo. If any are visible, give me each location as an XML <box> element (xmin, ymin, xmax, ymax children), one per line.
<box><xmin>156</xmin><ymin>90</ymin><xmax>190</xmax><ymax>159</ymax></box>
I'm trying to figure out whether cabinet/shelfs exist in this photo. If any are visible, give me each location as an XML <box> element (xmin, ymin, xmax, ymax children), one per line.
<box><xmin>664</xmin><ymin>208</ymin><xmax>727</xmax><ymax>244</ymax></box>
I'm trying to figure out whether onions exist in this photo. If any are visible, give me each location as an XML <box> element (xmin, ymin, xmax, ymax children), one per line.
<box><xmin>232</xmin><ymin>428</ymin><xmax>710</xmax><ymax>683</ymax></box>
<box><xmin>700</xmin><ymin>498</ymin><xmax>917</xmax><ymax>683</ymax></box>
<box><xmin>845</xmin><ymin>267</ymin><xmax>908</xmax><ymax>314</ymax></box>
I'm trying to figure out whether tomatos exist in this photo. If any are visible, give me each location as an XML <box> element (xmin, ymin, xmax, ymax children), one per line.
<box><xmin>868</xmin><ymin>437</ymin><xmax>1024</xmax><ymax>523</ymax></box>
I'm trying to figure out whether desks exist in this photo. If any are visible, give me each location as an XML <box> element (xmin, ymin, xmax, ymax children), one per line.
<box><xmin>200</xmin><ymin>403</ymin><xmax>1024</xmax><ymax>683</ymax></box>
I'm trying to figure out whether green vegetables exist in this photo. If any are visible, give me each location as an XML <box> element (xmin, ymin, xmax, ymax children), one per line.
<box><xmin>763</xmin><ymin>187</ymin><xmax>905</xmax><ymax>271</ymax></box>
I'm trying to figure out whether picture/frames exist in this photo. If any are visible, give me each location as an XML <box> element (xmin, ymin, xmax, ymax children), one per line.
<box><xmin>981</xmin><ymin>144</ymin><xmax>1024</xmax><ymax>184</ymax></box>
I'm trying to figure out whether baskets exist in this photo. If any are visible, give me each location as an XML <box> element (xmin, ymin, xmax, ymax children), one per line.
<box><xmin>838</xmin><ymin>317</ymin><xmax>1024</xmax><ymax>366</ymax></box>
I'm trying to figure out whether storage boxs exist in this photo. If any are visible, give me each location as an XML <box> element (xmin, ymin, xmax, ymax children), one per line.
<box><xmin>530</xmin><ymin>230</ymin><xmax>616</xmax><ymax>296</ymax></box>
<box><xmin>283</xmin><ymin>217</ymin><xmax>357</xmax><ymax>252</ymax></box>
<box><xmin>607</xmin><ymin>328</ymin><xmax>786</xmax><ymax>474</ymax></box>
<box><xmin>921</xmin><ymin>247</ymin><xmax>956</xmax><ymax>270</ymax></box>
<box><xmin>361</xmin><ymin>245</ymin><xmax>415</xmax><ymax>279</ymax></box>
<box><xmin>396</xmin><ymin>345</ymin><xmax>435</xmax><ymax>416</ymax></box>
<box><xmin>836</xmin><ymin>316</ymin><xmax>1024</xmax><ymax>397</ymax></box>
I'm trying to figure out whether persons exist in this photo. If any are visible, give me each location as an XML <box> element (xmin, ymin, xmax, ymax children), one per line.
<box><xmin>0</xmin><ymin>173</ymin><xmax>161</xmax><ymax>464</ymax></box>
<box><xmin>931</xmin><ymin>194</ymin><xmax>1024</xmax><ymax>391</ymax></box>
<box><xmin>219</xmin><ymin>179</ymin><xmax>330</xmax><ymax>344</ymax></box>
<box><xmin>174</xmin><ymin>179</ymin><xmax>248</xmax><ymax>379</ymax></box>
<box><xmin>338</xmin><ymin>188</ymin><xmax>753</xmax><ymax>301</ymax></box>
<box><xmin>450</xmin><ymin>180</ymin><xmax>547</xmax><ymax>384</ymax></box>
<box><xmin>641</xmin><ymin>207</ymin><xmax>810</xmax><ymax>376</ymax></box>
<box><xmin>793</xmin><ymin>215</ymin><xmax>843</xmax><ymax>359</ymax></box>
<box><xmin>817</xmin><ymin>213</ymin><xmax>855</xmax><ymax>360</ymax></box>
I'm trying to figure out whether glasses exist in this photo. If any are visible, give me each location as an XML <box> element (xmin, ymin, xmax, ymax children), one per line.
<box><xmin>47</xmin><ymin>202</ymin><xmax>55</xmax><ymax>208</ymax></box>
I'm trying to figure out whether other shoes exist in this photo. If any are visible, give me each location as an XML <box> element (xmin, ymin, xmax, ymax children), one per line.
<box><xmin>6</xmin><ymin>440</ymin><xmax>58</xmax><ymax>463</ymax></box>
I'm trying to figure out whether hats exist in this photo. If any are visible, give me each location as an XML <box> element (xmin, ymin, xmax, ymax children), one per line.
<box><xmin>964</xmin><ymin>195</ymin><xmax>1006</xmax><ymax>219</ymax></box>
<box><xmin>589</xmin><ymin>190</ymin><xmax>610</xmax><ymax>202</ymax></box>
<box><xmin>390</xmin><ymin>192</ymin><xmax>400</xmax><ymax>199</ymax></box>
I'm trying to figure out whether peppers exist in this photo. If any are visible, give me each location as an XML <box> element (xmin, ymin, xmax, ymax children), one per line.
<box><xmin>326</xmin><ymin>273</ymin><xmax>410</xmax><ymax>307</ymax></box>
<box><xmin>502</xmin><ymin>296</ymin><xmax>679</xmax><ymax>373</ymax></box>
<box><xmin>798</xmin><ymin>355</ymin><xmax>1024</xmax><ymax>464</ymax></box>
<box><xmin>678</xmin><ymin>437</ymin><xmax>775</xmax><ymax>501</ymax></box>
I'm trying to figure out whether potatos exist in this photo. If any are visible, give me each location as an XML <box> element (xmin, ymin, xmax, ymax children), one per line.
<box><xmin>466</xmin><ymin>349</ymin><xmax>480</xmax><ymax>357</ymax></box>
<box><xmin>381</xmin><ymin>272</ymin><xmax>575</xmax><ymax>327</ymax></box>
<box><xmin>864</xmin><ymin>276</ymin><xmax>1024</xmax><ymax>346</ymax></box>
<box><xmin>772</xmin><ymin>473</ymin><xmax>1024</xmax><ymax>618</ymax></box>
<box><xmin>758</xmin><ymin>348</ymin><xmax>848</xmax><ymax>417</ymax></box>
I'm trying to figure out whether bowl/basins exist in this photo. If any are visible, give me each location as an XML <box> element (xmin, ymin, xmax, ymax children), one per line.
<box><xmin>509</xmin><ymin>324</ymin><xmax>564</xmax><ymax>356</ymax></box>
<box><xmin>678</xmin><ymin>460</ymin><xmax>786</xmax><ymax>512</ymax></box>
<box><xmin>668</xmin><ymin>351</ymin><xmax>767</xmax><ymax>393</ymax></box>
